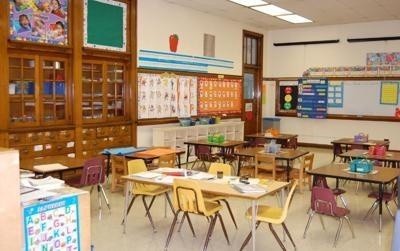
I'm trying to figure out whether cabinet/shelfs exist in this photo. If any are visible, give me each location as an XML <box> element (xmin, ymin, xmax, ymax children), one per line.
<box><xmin>152</xmin><ymin>120</ymin><xmax>246</xmax><ymax>167</ymax></box>
<box><xmin>0</xmin><ymin>0</ymin><xmax>76</xmax><ymax>188</ymax></box>
<box><xmin>0</xmin><ymin>144</ymin><xmax>90</xmax><ymax>250</ymax></box>
<box><xmin>73</xmin><ymin>0</ymin><xmax>139</xmax><ymax>155</ymax></box>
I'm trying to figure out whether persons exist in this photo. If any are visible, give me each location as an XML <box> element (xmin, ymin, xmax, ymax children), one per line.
<box><xmin>13</xmin><ymin>1</ymin><xmax>67</xmax><ymax>41</ymax></box>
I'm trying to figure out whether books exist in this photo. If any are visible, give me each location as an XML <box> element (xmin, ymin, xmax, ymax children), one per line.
<box><xmin>131</xmin><ymin>165</ymin><xmax>268</xmax><ymax>194</ymax></box>
<box><xmin>19</xmin><ymin>162</ymin><xmax>69</xmax><ymax>204</ymax></box>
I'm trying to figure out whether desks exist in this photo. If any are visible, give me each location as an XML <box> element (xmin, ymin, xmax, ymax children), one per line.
<box><xmin>106</xmin><ymin>145</ymin><xmax>187</xmax><ymax>193</ymax></box>
<box><xmin>183</xmin><ymin>130</ymin><xmax>400</xmax><ymax>233</ymax></box>
<box><xmin>117</xmin><ymin>166</ymin><xmax>291</xmax><ymax>250</ymax></box>
<box><xmin>21</xmin><ymin>155</ymin><xmax>85</xmax><ymax>181</ymax></box>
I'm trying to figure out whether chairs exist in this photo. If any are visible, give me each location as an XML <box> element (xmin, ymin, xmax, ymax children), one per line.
<box><xmin>118</xmin><ymin>159</ymin><xmax>179</xmax><ymax>234</ymax></box>
<box><xmin>237</xmin><ymin>179</ymin><xmax>298</xmax><ymax>250</ymax></box>
<box><xmin>63</xmin><ymin>157</ymin><xmax>111</xmax><ymax>213</ymax></box>
<box><xmin>163</xmin><ymin>179</ymin><xmax>232</xmax><ymax>250</ymax></box>
<box><xmin>300</xmin><ymin>185</ymin><xmax>356</xmax><ymax>250</ymax></box>
<box><xmin>175</xmin><ymin>161</ymin><xmax>239</xmax><ymax>238</ymax></box>
